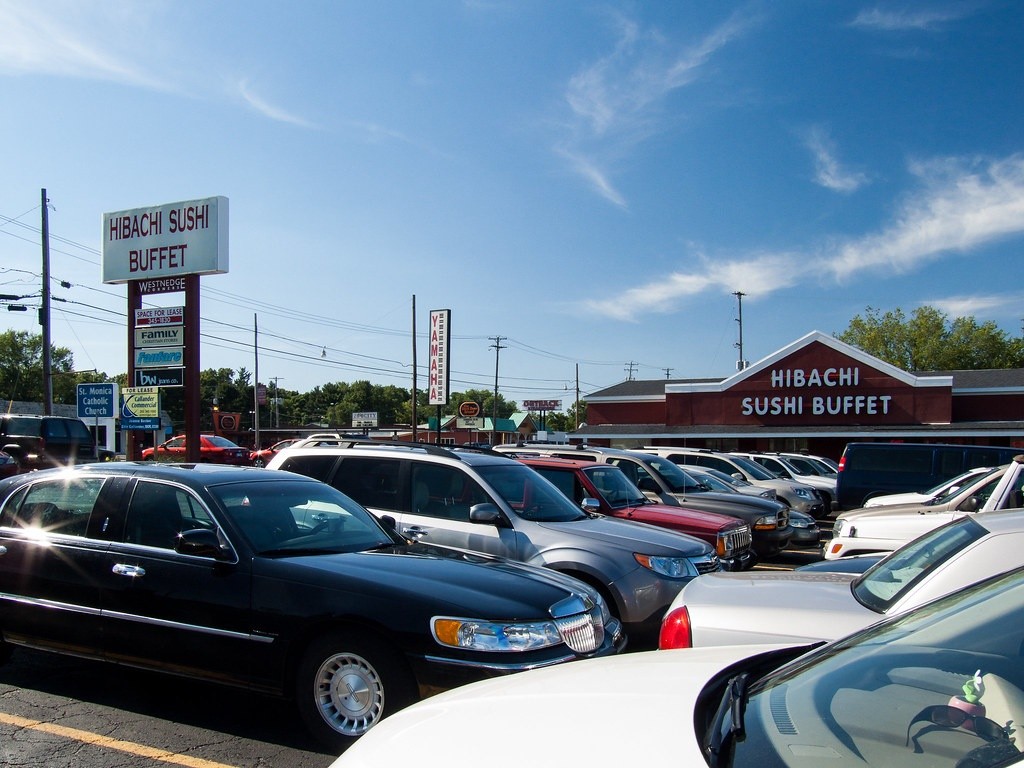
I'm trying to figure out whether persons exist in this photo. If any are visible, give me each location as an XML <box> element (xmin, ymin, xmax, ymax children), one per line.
<box><xmin>237</xmin><ymin>434</ymin><xmax>281</xmax><ymax>450</ymax></box>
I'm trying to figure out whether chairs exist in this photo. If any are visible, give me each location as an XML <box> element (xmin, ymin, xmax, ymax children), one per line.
<box><xmin>130</xmin><ymin>499</ymin><xmax>182</xmax><ymax>549</ymax></box>
<box><xmin>430</xmin><ymin>471</ymin><xmax>465</xmax><ymax>519</ymax></box>
<box><xmin>17</xmin><ymin>502</ymin><xmax>66</xmax><ymax>534</ymax></box>
<box><xmin>479</xmin><ymin>482</ymin><xmax>504</xmax><ymax>503</ymax></box>
<box><xmin>217</xmin><ymin>505</ymin><xmax>269</xmax><ymax>549</ymax></box>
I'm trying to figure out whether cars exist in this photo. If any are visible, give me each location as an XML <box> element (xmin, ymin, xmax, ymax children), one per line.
<box><xmin>141</xmin><ymin>435</ymin><xmax>250</xmax><ymax>467</ymax></box>
<box><xmin>327</xmin><ymin>563</ymin><xmax>1023</xmax><ymax>768</ymax></box>
<box><xmin>656</xmin><ymin>507</ymin><xmax>1023</xmax><ymax>651</ymax></box>
<box><xmin>611</xmin><ymin>445</ymin><xmax>841</xmax><ymax>517</ymax></box>
<box><xmin>250</xmin><ymin>439</ymin><xmax>304</xmax><ymax>468</ymax></box>
<box><xmin>463</xmin><ymin>442</ymin><xmax>479</xmax><ymax>453</ymax></box>
<box><xmin>0</xmin><ymin>461</ymin><xmax>615</xmax><ymax>746</ymax></box>
<box><xmin>863</xmin><ymin>467</ymin><xmax>995</xmax><ymax>507</ymax></box>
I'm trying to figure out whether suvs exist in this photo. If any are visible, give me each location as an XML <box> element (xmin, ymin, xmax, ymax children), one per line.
<box><xmin>0</xmin><ymin>413</ymin><xmax>98</xmax><ymax>480</ymax></box>
<box><xmin>491</xmin><ymin>440</ymin><xmax>794</xmax><ymax>558</ymax></box>
<box><xmin>822</xmin><ymin>460</ymin><xmax>1024</xmax><ymax>561</ymax></box>
<box><xmin>264</xmin><ymin>439</ymin><xmax>720</xmax><ymax>651</ymax></box>
<box><xmin>307</xmin><ymin>434</ymin><xmax>376</xmax><ymax>446</ymax></box>
<box><xmin>831</xmin><ymin>440</ymin><xmax>1024</xmax><ymax>511</ymax></box>
<box><xmin>428</xmin><ymin>456</ymin><xmax>759</xmax><ymax>574</ymax></box>
